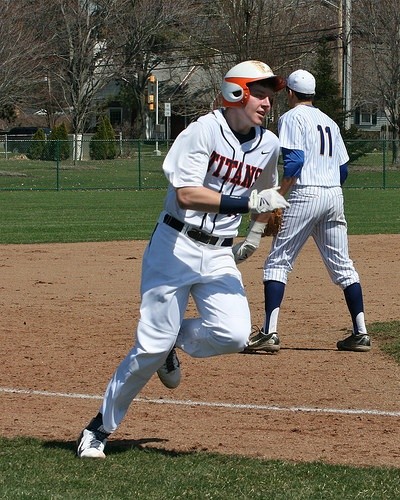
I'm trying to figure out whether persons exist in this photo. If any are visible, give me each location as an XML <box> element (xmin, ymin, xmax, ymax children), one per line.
<box><xmin>76</xmin><ymin>61</ymin><xmax>291</xmax><ymax>458</ymax></box>
<box><xmin>242</xmin><ymin>70</ymin><xmax>371</xmax><ymax>353</ymax></box>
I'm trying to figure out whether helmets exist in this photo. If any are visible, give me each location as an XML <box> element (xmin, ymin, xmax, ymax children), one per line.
<box><xmin>222</xmin><ymin>60</ymin><xmax>287</xmax><ymax>108</ymax></box>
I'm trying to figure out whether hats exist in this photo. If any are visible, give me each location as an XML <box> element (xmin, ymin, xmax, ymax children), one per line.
<box><xmin>287</xmin><ymin>69</ymin><xmax>316</xmax><ymax>95</ymax></box>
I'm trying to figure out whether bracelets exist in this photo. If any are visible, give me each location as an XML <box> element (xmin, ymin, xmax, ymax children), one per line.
<box><xmin>219</xmin><ymin>193</ymin><xmax>249</xmax><ymax>214</ymax></box>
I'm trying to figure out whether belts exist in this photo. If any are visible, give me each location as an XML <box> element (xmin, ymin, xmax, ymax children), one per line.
<box><xmin>164</xmin><ymin>214</ymin><xmax>233</xmax><ymax>247</ymax></box>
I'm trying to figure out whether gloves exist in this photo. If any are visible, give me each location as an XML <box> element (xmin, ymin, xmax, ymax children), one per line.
<box><xmin>249</xmin><ymin>186</ymin><xmax>291</xmax><ymax>213</ymax></box>
<box><xmin>232</xmin><ymin>239</ymin><xmax>257</xmax><ymax>265</ymax></box>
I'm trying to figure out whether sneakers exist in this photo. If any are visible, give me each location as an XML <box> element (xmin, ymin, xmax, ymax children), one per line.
<box><xmin>77</xmin><ymin>417</ymin><xmax>106</xmax><ymax>459</ymax></box>
<box><xmin>337</xmin><ymin>333</ymin><xmax>371</xmax><ymax>352</ymax></box>
<box><xmin>157</xmin><ymin>347</ymin><xmax>181</xmax><ymax>389</ymax></box>
<box><xmin>245</xmin><ymin>325</ymin><xmax>279</xmax><ymax>353</ymax></box>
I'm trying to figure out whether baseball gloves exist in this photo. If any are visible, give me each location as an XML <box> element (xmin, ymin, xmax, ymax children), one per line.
<box><xmin>261</xmin><ymin>209</ymin><xmax>285</xmax><ymax>238</ymax></box>
